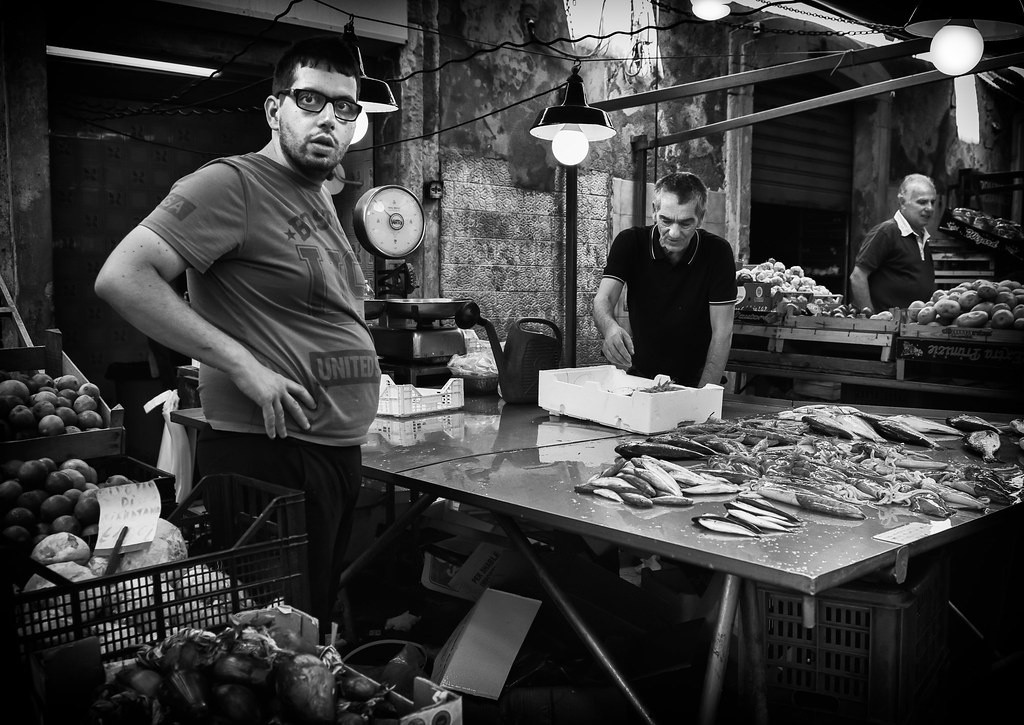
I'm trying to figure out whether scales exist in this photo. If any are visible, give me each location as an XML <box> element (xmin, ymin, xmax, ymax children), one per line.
<box><xmin>353</xmin><ymin>183</ymin><xmax>474</xmax><ymax>389</ymax></box>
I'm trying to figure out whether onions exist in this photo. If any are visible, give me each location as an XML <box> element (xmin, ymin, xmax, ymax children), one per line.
<box><xmin>0</xmin><ymin>373</ymin><xmax>133</xmax><ymax>546</ymax></box>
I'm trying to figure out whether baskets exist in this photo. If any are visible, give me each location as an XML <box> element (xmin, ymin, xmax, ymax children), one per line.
<box><xmin>1</xmin><ymin>470</ymin><xmax>316</xmax><ymax>719</ymax></box>
<box><xmin>734</xmin><ymin>548</ymin><xmax>954</xmax><ymax>724</ymax></box>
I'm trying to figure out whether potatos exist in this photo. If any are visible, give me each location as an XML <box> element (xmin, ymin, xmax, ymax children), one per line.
<box><xmin>910</xmin><ymin>279</ymin><xmax>1024</xmax><ymax>331</ymax></box>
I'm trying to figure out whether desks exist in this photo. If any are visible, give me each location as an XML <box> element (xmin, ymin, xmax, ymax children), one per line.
<box><xmin>170</xmin><ymin>400</ymin><xmax>1024</xmax><ymax>725</ymax></box>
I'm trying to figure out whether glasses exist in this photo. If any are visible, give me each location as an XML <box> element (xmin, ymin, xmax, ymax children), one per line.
<box><xmin>275</xmin><ymin>88</ymin><xmax>363</xmax><ymax>123</ymax></box>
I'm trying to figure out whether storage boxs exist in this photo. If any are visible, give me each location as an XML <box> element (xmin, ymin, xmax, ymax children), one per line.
<box><xmin>0</xmin><ymin>472</ymin><xmax>308</xmax><ymax>672</ymax></box>
<box><xmin>538</xmin><ymin>364</ymin><xmax>724</xmax><ymax>435</ymax></box>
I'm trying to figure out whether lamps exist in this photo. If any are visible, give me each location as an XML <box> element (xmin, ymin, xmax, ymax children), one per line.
<box><xmin>341</xmin><ymin>14</ymin><xmax>399</xmax><ymax>145</ymax></box>
<box><xmin>529</xmin><ymin>60</ymin><xmax>617</xmax><ymax>165</ymax></box>
<box><xmin>904</xmin><ymin>0</ymin><xmax>1024</xmax><ymax>77</ymax></box>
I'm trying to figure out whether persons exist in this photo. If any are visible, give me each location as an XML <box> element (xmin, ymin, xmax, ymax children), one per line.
<box><xmin>95</xmin><ymin>37</ymin><xmax>382</xmax><ymax>638</ymax></box>
<box><xmin>849</xmin><ymin>173</ymin><xmax>938</xmax><ymax>313</ymax></box>
<box><xmin>592</xmin><ymin>172</ymin><xmax>738</xmax><ymax>388</ymax></box>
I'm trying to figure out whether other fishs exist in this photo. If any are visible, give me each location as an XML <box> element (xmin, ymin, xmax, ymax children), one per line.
<box><xmin>614</xmin><ymin>442</ymin><xmax>704</xmax><ymax>462</ymax></box>
<box><xmin>779</xmin><ymin>401</ymin><xmax>1024</xmax><ymax>463</ymax></box>
<box><xmin>679</xmin><ymin>411</ymin><xmax>778</xmax><ymax>432</ymax></box>
<box><xmin>569</xmin><ymin>455</ymin><xmax>801</xmax><ymax>537</ymax></box>
<box><xmin>647</xmin><ymin>434</ymin><xmax>720</xmax><ymax>456</ymax></box>
